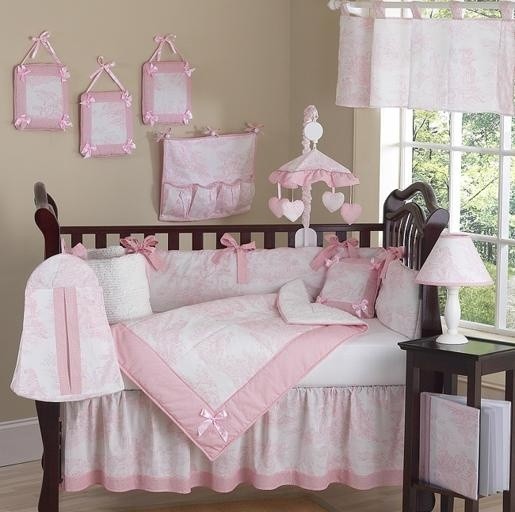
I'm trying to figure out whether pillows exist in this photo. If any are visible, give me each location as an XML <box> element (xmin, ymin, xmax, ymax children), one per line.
<box><xmin>78</xmin><ymin>253</ymin><xmax>154</xmax><ymax>325</ymax></box>
<box><xmin>314</xmin><ymin>257</ymin><xmax>385</xmax><ymax>320</ymax></box>
<box><xmin>374</xmin><ymin>258</ymin><xmax>423</xmax><ymax>340</ymax></box>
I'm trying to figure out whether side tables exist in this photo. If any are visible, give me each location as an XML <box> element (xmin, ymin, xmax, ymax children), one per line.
<box><xmin>397</xmin><ymin>333</ymin><xmax>515</xmax><ymax>512</ymax></box>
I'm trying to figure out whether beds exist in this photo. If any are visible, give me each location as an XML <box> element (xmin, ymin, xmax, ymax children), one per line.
<box><xmin>31</xmin><ymin>180</ymin><xmax>451</xmax><ymax>511</ymax></box>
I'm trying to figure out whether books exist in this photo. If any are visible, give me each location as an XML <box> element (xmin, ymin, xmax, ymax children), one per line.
<box><xmin>420</xmin><ymin>392</ymin><xmax>511</xmax><ymax>500</ymax></box>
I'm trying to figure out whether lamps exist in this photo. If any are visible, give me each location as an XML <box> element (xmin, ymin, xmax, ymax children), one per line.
<box><xmin>415</xmin><ymin>234</ymin><xmax>496</xmax><ymax>347</ymax></box>
